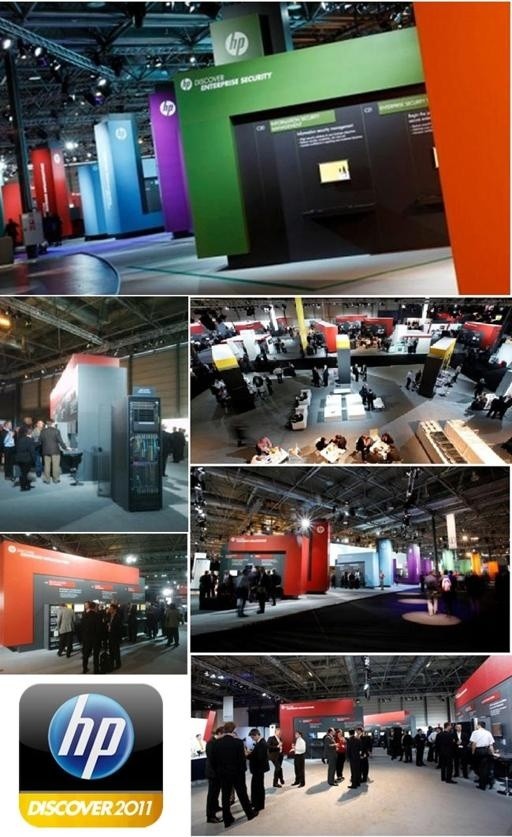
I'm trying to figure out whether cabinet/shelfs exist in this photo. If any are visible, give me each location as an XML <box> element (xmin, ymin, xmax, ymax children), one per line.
<box><xmin>44</xmin><ymin>601</ymin><xmax>148</xmax><ymax>650</ymax></box>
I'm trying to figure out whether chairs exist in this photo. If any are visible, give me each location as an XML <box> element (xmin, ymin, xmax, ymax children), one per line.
<box><xmin>251</xmin><ymin>428</ymin><xmax>404</xmax><ymax>464</ymax></box>
<box><xmin>493</xmin><ymin>753</ymin><xmax>512</xmax><ymax>796</ymax></box>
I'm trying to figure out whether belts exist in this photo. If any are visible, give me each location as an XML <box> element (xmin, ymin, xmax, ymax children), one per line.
<box><xmin>477</xmin><ymin>747</ymin><xmax>490</xmax><ymax>748</ymax></box>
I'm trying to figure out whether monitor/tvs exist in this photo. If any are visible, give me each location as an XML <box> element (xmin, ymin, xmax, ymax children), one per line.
<box><xmin>318</xmin><ymin>158</ymin><xmax>352</xmax><ymax>185</ymax></box>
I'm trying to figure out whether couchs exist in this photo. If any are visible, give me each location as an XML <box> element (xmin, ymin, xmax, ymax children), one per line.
<box><xmin>292</xmin><ymin>388</ymin><xmax>385</xmax><ymax>431</ymax></box>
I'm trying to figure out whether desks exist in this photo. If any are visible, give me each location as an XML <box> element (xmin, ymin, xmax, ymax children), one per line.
<box><xmin>62</xmin><ymin>448</ymin><xmax>86</xmax><ymax>486</ymax></box>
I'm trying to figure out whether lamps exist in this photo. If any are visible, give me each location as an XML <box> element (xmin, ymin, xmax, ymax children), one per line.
<box><xmin>287</xmin><ymin>2</ymin><xmax>302</xmax><ymax>10</ymax></box>
<box><xmin>145</xmin><ymin>55</ymin><xmax>164</xmax><ymax>69</ymax></box>
<box><xmin>2</xmin><ymin>37</ymin><xmax>108</xmax><ymax>100</ymax></box>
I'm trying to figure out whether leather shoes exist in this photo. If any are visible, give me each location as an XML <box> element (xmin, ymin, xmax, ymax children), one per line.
<box><xmin>207</xmin><ymin>777</ymin><xmax>367</xmax><ymax>827</ymax></box>
<box><xmin>391</xmin><ymin>756</ymin><xmax>493</xmax><ymax>790</ymax></box>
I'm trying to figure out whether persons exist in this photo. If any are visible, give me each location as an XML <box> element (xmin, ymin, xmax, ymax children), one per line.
<box><xmin>42</xmin><ymin>211</ymin><xmax>53</xmax><ymax>247</ymax></box>
<box><xmin>330</xmin><ymin>569</ymin><xmax>385</xmax><ymax>591</ymax></box>
<box><xmin>254</xmin><ymin>433</ymin><xmax>399</xmax><ymax>464</ymax></box>
<box><xmin>4</xmin><ymin>216</ymin><xmax>22</xmax><ymax>253</ymax></box>
<box><xmin>193</xmin><ymin>720</ymin><xmax>501</xmax><ymax>828</ymax></box>
<box><xmin>54</xmin><ymin>600</ymin><xmax>183</xmax><ymax>672</ymax></box>
<box><xmin>190</xmin><ymin>321</ymin><xmax>512</xmax><ymax>421</ymax></box>
<box><xmin>413</xmin><ymin>568</ymin><xmax>508</xmax><ymax>619</ymax></box>
<box><xmin>202</xmin><ymin>567</ymin><xmax>283</xmax><ymax>616</ymax></box>
<box><xmin>54</xmin><ymin>214</ymin><xmax>64</xmax><ymax>247</ymax></box>
<box><xmin>0</xmin><ymin>414</ymin><xmax>64</xmax><ymax>491</ymax></box>
